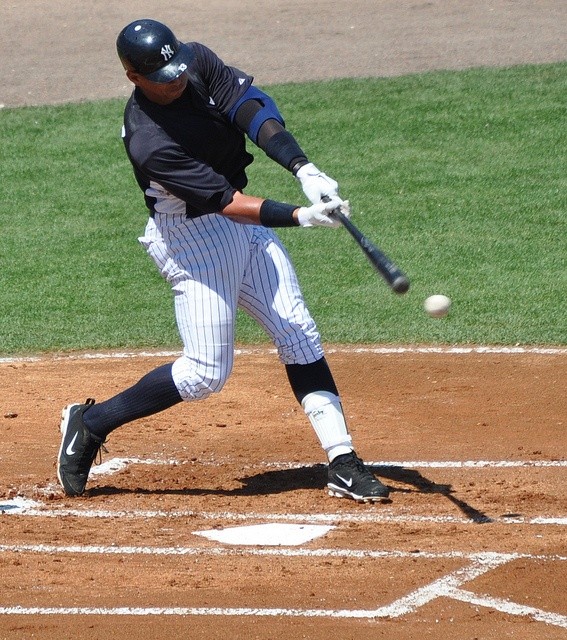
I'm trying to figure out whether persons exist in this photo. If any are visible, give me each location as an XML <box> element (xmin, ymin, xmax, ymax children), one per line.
<box><xmin>57</xmin><ymin>19</ymin><xmax>391</xmax><ymax>502</ymax></box>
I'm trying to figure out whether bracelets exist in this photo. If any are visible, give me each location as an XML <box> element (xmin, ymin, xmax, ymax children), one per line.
<box><xmin>291</xmin><ymin>158</ymin><xmax>310</xmax><ymax>176</ymax></box>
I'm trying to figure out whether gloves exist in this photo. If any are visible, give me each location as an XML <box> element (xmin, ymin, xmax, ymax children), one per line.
<box><xmin>295</xmin><ymin>161</ymin><xmax>339</xmax><ymax>206</ymax></box>
<box><xmin>296</xmin><ymin>198</ymin><xmax>350</xmax><ymax>229</ymax></box>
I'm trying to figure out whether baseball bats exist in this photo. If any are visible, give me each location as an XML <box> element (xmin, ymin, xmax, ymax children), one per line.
<box><xmin>323</xmin><ymin>196</ymin><xmax>410</xmax><ymax>296</ymax></box>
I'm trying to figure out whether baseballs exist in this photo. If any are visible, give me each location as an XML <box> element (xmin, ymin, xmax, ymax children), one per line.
<box><xmin>422</xmin><ymin>294</ymin><xmax>453</xmax><ymax>318</ymax></box>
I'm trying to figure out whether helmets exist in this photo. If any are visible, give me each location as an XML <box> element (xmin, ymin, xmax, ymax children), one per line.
<box><xmin>115</xmin><ymin>19</ymin><xmax>196</xmax><ymax>84</ymax></box>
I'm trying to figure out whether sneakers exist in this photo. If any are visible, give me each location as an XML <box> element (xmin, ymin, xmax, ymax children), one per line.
<box><xmin>55</xmin><ymin>397</ymin><xmax>110</xmax><ymax>497</ymax></box>
<box><xmin>326</xmin><ymin>447</ymin><xmax>389</xmax><ymax>503</ymax></box>
